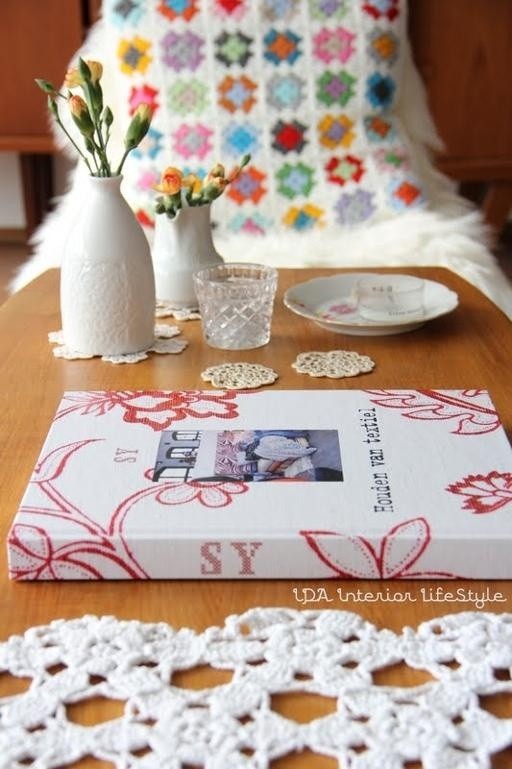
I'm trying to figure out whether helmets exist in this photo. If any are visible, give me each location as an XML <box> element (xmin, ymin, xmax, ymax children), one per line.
<box><xmin>283</xmin><ymin>272</ymin><xmax>458</xmax><ymax>340</ymax></box>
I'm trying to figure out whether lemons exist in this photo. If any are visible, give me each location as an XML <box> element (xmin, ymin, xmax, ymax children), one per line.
<box><xmin>147</xmin><ymin>196</ymin><xmax>225</xmax><ymax>307</ymax></box>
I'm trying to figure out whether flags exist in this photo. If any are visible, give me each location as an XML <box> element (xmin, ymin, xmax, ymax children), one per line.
<box><xmin>57</xmin><ymin>175</ymin><xmax>224</xmax><ymax>361</ymax></box>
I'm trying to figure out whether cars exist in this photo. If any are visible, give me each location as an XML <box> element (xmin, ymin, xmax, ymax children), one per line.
<box><xmin>194</xmin><ymin>264</ymin><xmax>279</xmax><ymax>352</ymax></box>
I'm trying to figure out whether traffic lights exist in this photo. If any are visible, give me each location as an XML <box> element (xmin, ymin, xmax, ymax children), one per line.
<box><xmin>104</xmin><ymin>0</ymin><xmax>433</xmax><ymax>236</ymax></box>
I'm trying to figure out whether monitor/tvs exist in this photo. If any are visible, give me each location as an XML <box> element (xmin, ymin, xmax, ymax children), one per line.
<box><xmin>7</xmin><ymin>389</ymin><xmax>512</xmax><ymax>580</ymax></box>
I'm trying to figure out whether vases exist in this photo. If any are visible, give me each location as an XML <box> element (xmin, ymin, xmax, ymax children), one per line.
<box><xmin>31</xmin><ymin>59</ymin><xmax>252</xmax><ymax>217</ymax></box>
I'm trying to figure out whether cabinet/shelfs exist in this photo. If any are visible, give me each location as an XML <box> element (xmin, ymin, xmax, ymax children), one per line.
<box><xmin>58</xmin><ymin>171</ymin><xmax>155</xmax><ymax>356</ymax></box>
